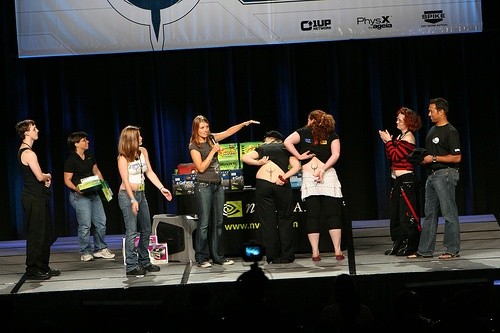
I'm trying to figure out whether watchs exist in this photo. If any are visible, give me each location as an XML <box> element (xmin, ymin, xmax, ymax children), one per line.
<box><xmin>432</xmin><ymin>155</ymin><xmax>436</xmax><ymax>162</ymax></box>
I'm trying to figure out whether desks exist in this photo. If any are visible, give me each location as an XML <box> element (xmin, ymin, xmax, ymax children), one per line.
<box><xmin>174</xmin><ymin>187</ymin><xmax>353</xmax><ymax>257</ymax></box>
<box><xmin>152</xmin><ymin>214</ymin><xmax>199</xmax><ymax>263</ymax></box>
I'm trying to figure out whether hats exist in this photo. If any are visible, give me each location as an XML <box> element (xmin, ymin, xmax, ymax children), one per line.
<box><xmin>266</xmin><ymin>131</ymin><xmax>283</xmax><ymax>139</ymax></box>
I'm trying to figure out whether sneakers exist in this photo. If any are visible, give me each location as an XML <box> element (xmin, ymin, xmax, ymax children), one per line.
<box><xmin>94</xmin><ymin>248</ymin><xmax>116</xmax><ymax>259</ymax></box>
<box><xmin>80</xmin><ymin>253</ymin><xmax>94</xmax><ymax>261</ymax></box>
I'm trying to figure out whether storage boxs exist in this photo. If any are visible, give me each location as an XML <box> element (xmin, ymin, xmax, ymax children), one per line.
<box><xmin>77</xmin><ymin>175</ymin><xmax>114</xmax><ymax>202</ymax></box>
<box><xmin>219</xmin><ymin>170</ymin><xmax>245</xmax><ymax>189</ymax></box>
<box><xmin>217</xmin><ymin>142</ymin><xmax>265</xmax><ymax>170</ymax></box>
<box><xmin>172</xmin><ymin>163</ymin><xmax>198</xmax><ymax>195</ymax></box>
<box><xmin>288</xmin><ymin>163</ymin><xmax>303</xmax><ymax>187</ymax></box>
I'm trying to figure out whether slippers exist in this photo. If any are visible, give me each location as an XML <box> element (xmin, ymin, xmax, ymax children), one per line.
<box><xmin>407</xmin><ymin>252</ymin><xmax>433</xmax><ymax>259</ymax></box>
<box><xmin>439</xmin><ymin>252</ymin><xmax>460</xmax><ymax>259</ymax></box>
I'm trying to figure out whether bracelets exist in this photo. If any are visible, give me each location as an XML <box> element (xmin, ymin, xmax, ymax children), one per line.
<box><xmin>320</xmin><ymin>169</ymin><xmax>326</xmax><ymax>173</ymax></box>
<box><xmin>278</xmin><ymin>175</ymin><xmax>287</xmax><ymax>182</ymax></box>
<box><xmin>159</xmin><ymin>187</ymin><xmax>164</xmax><ymax>191</ymax></box>
<box><xmin>130</xmin><ymin>198</ymin><xmax>136</xmax><ymax>203</ymax></box>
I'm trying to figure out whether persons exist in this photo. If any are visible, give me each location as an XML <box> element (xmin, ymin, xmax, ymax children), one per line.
<box><xmin>378</xmin><ymin>107</ymin><xmax>422</xmax><ymax>255</ymax></box>
<box><xmin>63</xmin><ymin>132</ymin><xmax>115</xmax><ymax>262</ymax></box>
<box><xmin>407</xmin><ymin>98</ymin><xmax>462</xmax><ymax>259</ymax></box>
<box><xmin>14</xmin><ymin>120</ymin><xmax>61</xmax><ymax>281</ymax></box>
<box><xmin>118</xmin><ymin>125</ymin><xmax>172</xmax><ymax>275</ymax></box>
<box><xmin>189</xmin><ymin>116</ymin><xmax>255</xmax><ymax>268</ymax></box>
<box><xmin>242</xmin><ymin>130</ymin><xmax>302</xmax><ymax>264</ymax></box>
<box><xmin>284</xmin><ymin>110</ymin><xmax>344</xmax><ymax>262</ymax></box>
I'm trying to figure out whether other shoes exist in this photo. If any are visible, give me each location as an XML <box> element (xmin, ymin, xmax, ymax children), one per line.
<box><xmin>26</xmin><ymin>266</ymin><xmax>61</xmax><ymax>280</ymax></box>
<box><xmin>126</xmin><ymin>266</ymin><xmax>146</xmax><ymax>275</ymax></box>
<box><xmin>215</xmin><ymin>258</ymin><xmax>234</xmax><ymax>264</ymax></box>
<box><xmin>335</xmin><ymin>254</ymin><xmax>345</xmax><ymax>260</ymax></box>
<box><xmin>144</xmin><ymin>264</ymin><xmax>161</xmax><ymax>271</ymax></box>
<box><xmin>197</xmin><ymin>261</ymin><xmax>211</xmax><ymax>268</ymax></box>
<box><xmin>312</xmin><ymin>255</ymin><xmax>320</xmax><ymax>261</ymax></box>
<box><xmin>385</xmin><ymin>240</ymin><xmax>418</xmax><ymax>256</ymax></box>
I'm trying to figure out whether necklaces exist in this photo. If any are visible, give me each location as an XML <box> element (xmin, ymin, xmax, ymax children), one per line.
<box><xmin>134</xmin><ymin>155</ymin><xmax>142</xmax><ymax>167</ymax></box>
<box><xmin>23</xmin><ymin>142</ymin><xmax>31</xmax><ymax>148</ymax></box>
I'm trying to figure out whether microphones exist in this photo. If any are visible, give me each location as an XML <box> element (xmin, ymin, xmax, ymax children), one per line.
<box><xmin>209</xmin><ymin>135</ymin><xmax>216</xmax><ymax>144</ymax></box>
